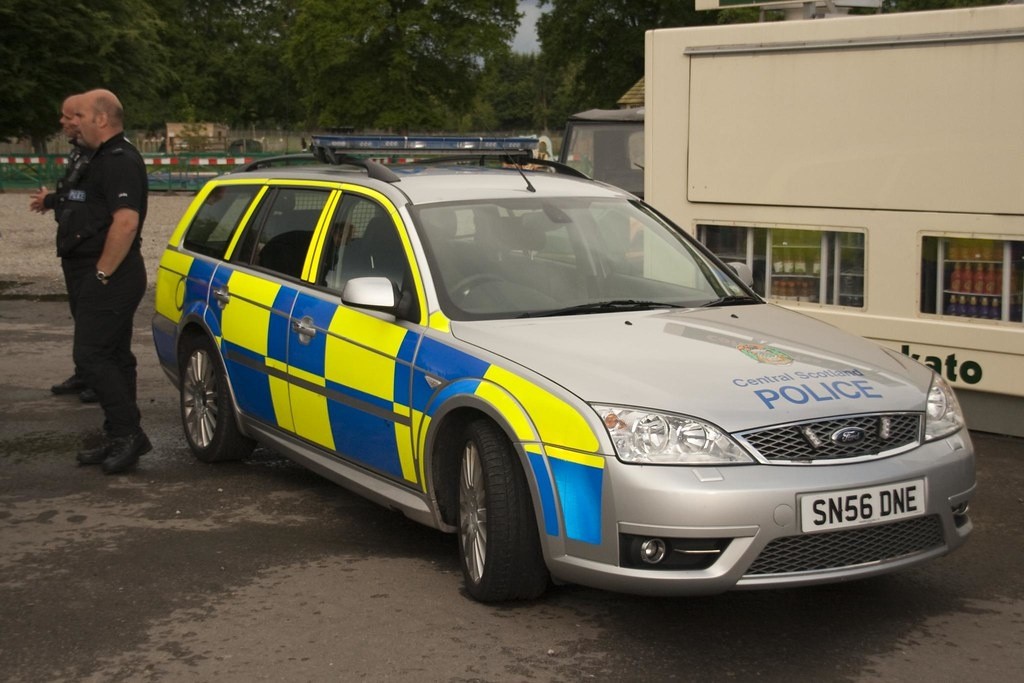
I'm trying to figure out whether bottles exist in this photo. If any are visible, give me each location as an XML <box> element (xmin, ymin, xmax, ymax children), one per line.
<box><xmin>947</xmin><ymin>239</ymin><xmax>1021</xmax><ymax>323</ymax></box>
<box><xmin>770</xmin><ymin>232</ymin><xmax>822</xmax><ymax>302</ymax></box>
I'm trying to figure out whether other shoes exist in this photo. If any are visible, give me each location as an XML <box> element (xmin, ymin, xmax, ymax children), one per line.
<box><xmin>51</xmin><ymin>374</ymin><xmax>84</xmax><ymax>394</ymax></box>
<box><xmin>103</xmin><ymin>429</ymin><xmax>153</xmax><ymax>473</ymax></box>
<box><xmin>76</xmin><ymin>434</ymin><xmax>124</xmax><ymax>464</ymax></box>
<box><xmin>80</xmin><ymin>388</ymin><xmax>99</xmax><ymax>404</ymax></box>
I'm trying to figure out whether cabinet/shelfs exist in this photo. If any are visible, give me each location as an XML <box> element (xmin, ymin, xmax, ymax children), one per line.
<box><xmin>931</xmin><ymin>237</ymin><xmax>1024</xmax><ymax>322</ymax></box>
<box><xmin>699</xmin><ymin>225</ymin><xmax>864</xmax><ymax>306</ymax></box>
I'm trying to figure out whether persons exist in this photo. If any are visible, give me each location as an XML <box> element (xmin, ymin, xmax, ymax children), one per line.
<box><xmin>26</xmin><ymin>89</ymin><xmax>152</xmax><ymax>475</ymax></box>
<box><xmin>155</xmin><ymin>132</ymin><xmax>165</xmax><ymax>151</ymax></box>
<box><xmin>503</xmin><ymin>141</ymin><xmax>550</xmax><ymax>172</ymax></box>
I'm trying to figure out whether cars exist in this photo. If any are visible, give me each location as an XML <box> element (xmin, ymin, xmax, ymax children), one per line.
<box><xmin>150</xmin><ymin>134</ymin><xmax>979</xmax><ymax>607</ymax></box>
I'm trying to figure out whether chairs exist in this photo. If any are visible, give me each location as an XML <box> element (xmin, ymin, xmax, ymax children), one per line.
<box><xmin>481</xmin><ymin>214</ymin><xmax>560</xmax><ymax>302</ymax></box>
<box><xmin>358</xmin><ymin>217</ymin><xmax>452</xmax><ymax>294</ymax></box>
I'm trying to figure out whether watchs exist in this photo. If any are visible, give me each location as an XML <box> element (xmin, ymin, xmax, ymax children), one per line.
<box><xmin>95</xmin><ymin>269</ymin><xmax>110</xmax><ymax>281</ymax></box>
